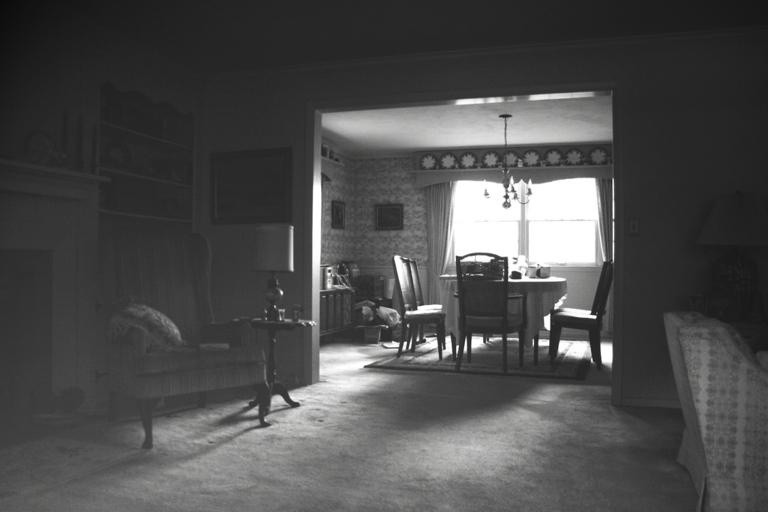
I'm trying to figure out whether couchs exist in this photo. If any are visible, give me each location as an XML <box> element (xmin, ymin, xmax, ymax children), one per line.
<box><xmin>104</xmin><ymin>315</ymin><xmax>271</xmax><ymax>449</ymax></box>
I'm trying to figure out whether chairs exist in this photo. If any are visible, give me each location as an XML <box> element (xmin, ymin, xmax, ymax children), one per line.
<box><xmin>393</xmin><ymin>253</ymin><xmax>614</xmax><ymax>377</ymax></box>
<box><xmin>665</xmin><ymin>308</ymin><xmax>768</xmax><ymax>512</ymax></box>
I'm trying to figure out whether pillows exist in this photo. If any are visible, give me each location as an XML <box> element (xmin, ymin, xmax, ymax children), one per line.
<box><xmin>123</xmin><ymin>304</ymin><xmax>183</xmax><ymax>348</ymax></box>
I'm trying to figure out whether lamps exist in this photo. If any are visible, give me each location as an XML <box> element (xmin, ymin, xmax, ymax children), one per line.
<box><xmin>485</xmin><ymin>114</ymin><xmax>534</xmax><ymax>208</ymax></box>
<box><xmin>254</xmin><ymin>223</ymin><xmax>295</xmax><ymax>318</ymax></box>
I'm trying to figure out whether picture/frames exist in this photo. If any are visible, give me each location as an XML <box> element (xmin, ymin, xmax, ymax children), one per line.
<box><xmin>330</xmin><ymin>199</ymin><xmax>348</xmax><ymax>230</ymax></box>
<box><xmin>211</xmin><ymin>144</ymin><xmax>293</xmax><ymax>225</ymax></box>
<box><xmin>373</xmin><ymin>202</ymin><xmax>405</xmax><ymax>230</ymax></box>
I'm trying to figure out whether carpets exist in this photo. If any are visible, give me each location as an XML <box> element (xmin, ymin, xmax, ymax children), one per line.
<box><xmin>366</xmin><ymin>337</ymin><xmax>604</xmax><ymax>382</ymax></box>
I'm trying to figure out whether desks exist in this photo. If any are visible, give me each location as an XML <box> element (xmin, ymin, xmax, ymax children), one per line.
<box><xmin>248</xmin><ymin>317</ymin><xmax>314</xmax><ymax>428</ymax></box>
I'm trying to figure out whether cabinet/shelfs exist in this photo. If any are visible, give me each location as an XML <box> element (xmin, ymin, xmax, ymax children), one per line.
<box><xmin>321</xmin><ymin>291</ymin><xmax>352</xmax><ymax>338</ymax></box>
<box><xmin>71</xmin><ymin>80</ymin><xmax>197</xmax><ymax>223</ymax></box>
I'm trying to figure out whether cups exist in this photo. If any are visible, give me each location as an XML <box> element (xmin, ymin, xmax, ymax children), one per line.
<box><xmin>278</xmin><ymin>309</ymin><xmax>285</xmax><ymax>322</ymax></box>
